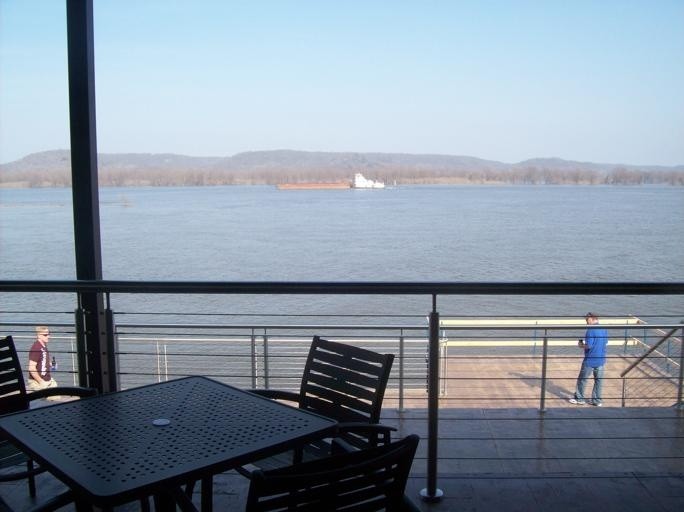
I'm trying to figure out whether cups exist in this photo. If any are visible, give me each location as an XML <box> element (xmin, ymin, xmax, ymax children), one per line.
<box><xmin>579</xmin><ymin>340</ymin><xmax>583</xmax><ymax>343</ymax></box>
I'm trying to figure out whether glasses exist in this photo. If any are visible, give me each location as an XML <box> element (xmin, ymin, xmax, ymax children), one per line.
<box><xmin>40</xmin><ymin>334</ymin><xmax>50</xmax><ymax>337</ymax></box>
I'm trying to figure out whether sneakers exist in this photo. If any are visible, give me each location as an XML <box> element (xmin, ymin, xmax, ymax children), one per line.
<box><xmin>568</xmin><ymin>398</ymin><xmax>586</xmax><ymax>405</ymax></box>
<box><xmin>587</xmin><ymin>398</ymin><xmax>602</xmax><ymax>407</ymax></box>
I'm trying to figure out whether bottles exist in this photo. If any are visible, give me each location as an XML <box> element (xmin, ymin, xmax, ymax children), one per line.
<box><xmin>51</xmin><ymin>356</ymin><xmax>56</xmax><ymax>371</ymax></box>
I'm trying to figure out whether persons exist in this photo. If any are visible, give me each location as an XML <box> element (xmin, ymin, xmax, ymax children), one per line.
<box><xmin>568</xmin><ymin>312</ymin><xmax>609</xmax><ymax>407</ymax></box>
<box><xmin>27</xmin><ymin>326</ymin><xmax>62</xmax><ymax>401</ymax></box>
